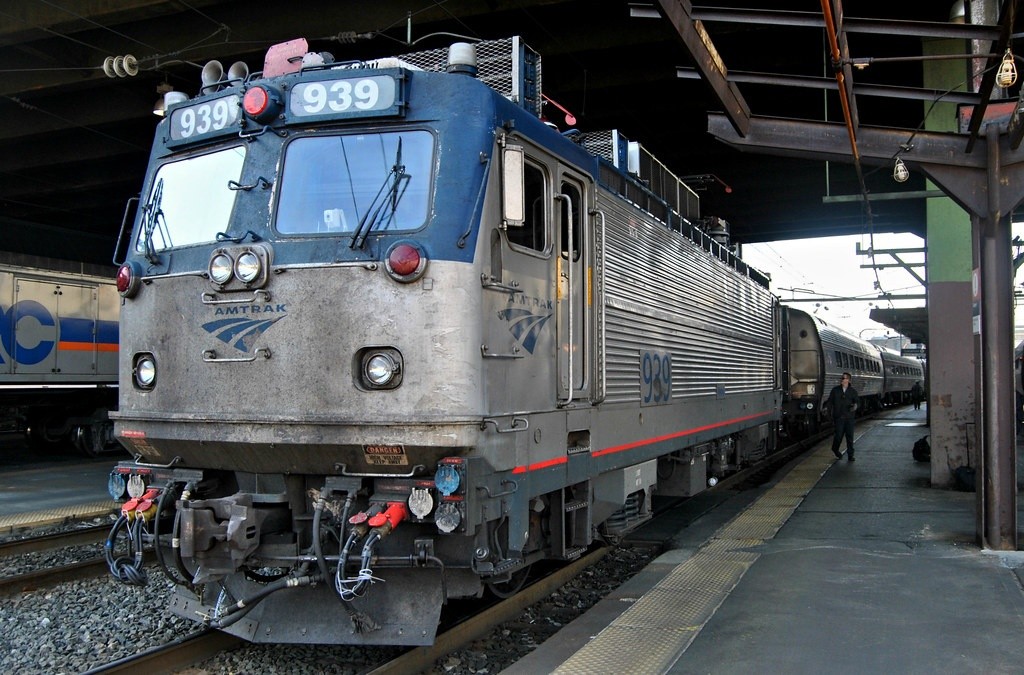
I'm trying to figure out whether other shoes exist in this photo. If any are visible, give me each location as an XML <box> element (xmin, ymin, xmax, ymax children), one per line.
<box><xmin>848</xmin><ymin>456</ymin><xmax>856</xmax><ymax>461</ymax></box>
<box><xmin>834</xmin><ymin>450</ymin><xmax>842</xmax><ymax>457</ymax></box>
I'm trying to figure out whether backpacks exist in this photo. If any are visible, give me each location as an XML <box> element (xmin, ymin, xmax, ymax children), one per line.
<box><xmin>951</xmin><ymin>466</ymin><xmax>977</xmax><ymax>492</ymax></box>
<box><xmin>912</xmin><ymin>435</ymin><xmax>931</xmax><ymax>463</ymax></box>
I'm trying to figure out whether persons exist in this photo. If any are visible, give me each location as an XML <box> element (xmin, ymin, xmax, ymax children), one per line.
<box><xmin>911</xmin><ymin>381</ymin><xmax>924</xmax><ymax>410</ymax></box>
<box><xmin>827</xmin><ymin>372</ymin><xmax>860</xmax><ymax>461</ymax></box>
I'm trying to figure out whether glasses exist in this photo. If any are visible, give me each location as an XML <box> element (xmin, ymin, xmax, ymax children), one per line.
<box><xmin>841</xmin><ymin>377</ymin><xmax>849</xmax><ymax>380</ymax></box>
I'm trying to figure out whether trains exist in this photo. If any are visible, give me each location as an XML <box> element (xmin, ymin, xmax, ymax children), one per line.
<box><xmin>0</xmin><ymin>264</ymin><xmax>120</xmax><ymax>461</ymax></box>
<box><xmin>106</xmin><ymin>36</ymin><xmax>928</xmax><ymax>646</ymax></box>
